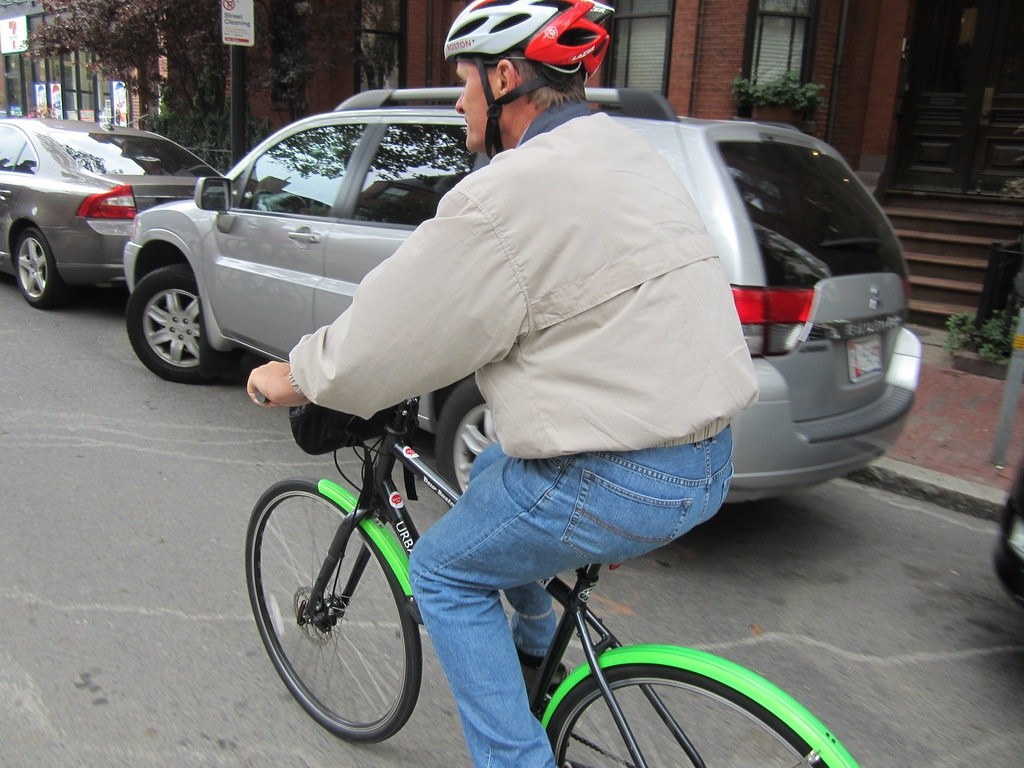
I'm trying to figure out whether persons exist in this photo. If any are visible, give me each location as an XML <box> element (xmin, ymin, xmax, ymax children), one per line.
<box><xmin>246</xmin><ymin>0</ymin><xmax>761</xmax><ymax>768</ymax></box>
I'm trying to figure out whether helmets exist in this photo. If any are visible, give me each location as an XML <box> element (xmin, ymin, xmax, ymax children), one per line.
<box><xmin>443</xmin><ymin>0</ymin><xmax>618</xmax><ymax>76</ymax></box>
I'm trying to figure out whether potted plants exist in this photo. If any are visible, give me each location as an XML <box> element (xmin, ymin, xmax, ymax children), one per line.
<box><xmin>732</xmin><ymin>70</ymin><xmax>826</xmax><ymax>123</ymax></box>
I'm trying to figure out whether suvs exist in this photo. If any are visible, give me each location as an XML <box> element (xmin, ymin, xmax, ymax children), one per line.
<box><xmin>122</xmin><ymin>87</ymin><xmax>921</xmax><ymax>516</ymax></box>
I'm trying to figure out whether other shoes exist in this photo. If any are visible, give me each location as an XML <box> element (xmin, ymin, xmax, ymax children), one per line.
<box><xmin>517</xmin><ymin>655</ymin><xmax>570</xmax><ymax>714</ymax></box>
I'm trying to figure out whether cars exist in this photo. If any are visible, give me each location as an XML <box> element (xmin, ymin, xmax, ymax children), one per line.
<box><xmin>1</xmin><ymin>117</ymin><xmax>230</xmax><ymax>309</ymax></box>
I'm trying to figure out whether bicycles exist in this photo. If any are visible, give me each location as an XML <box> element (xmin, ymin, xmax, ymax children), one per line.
<box><xmin>242</xmin><ymin>379</ymin><xmax>864</xmax><ymax>768</ymax></box>
